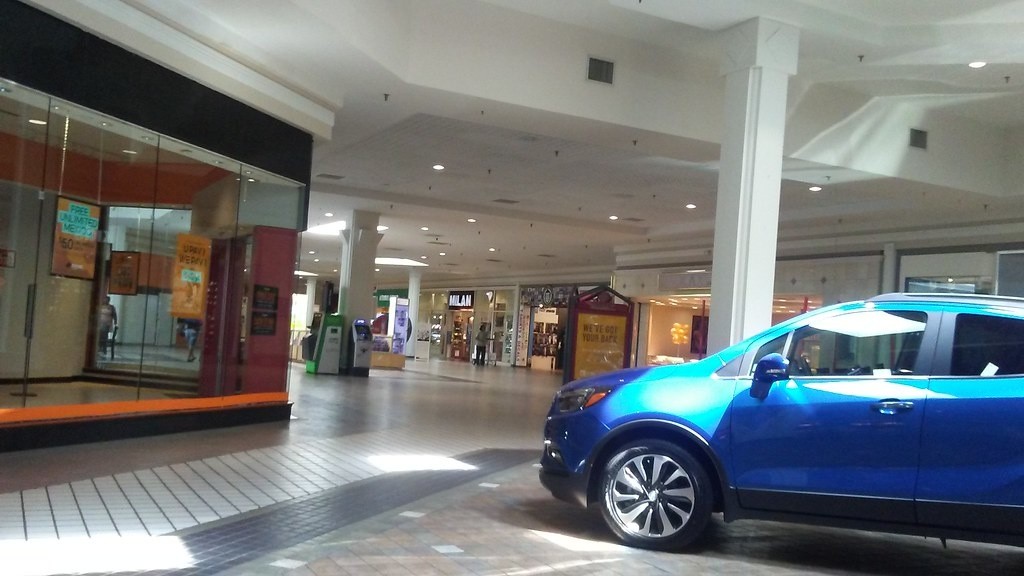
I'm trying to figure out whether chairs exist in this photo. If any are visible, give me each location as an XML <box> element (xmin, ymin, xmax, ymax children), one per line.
<box><xmin>107</xmin><ymin>326</ymin><xmax>119</xmax><ymax>360</ymax></box>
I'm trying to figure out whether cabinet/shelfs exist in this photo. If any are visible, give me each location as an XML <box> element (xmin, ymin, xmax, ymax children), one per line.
<box><xmin>289</xmin><ymin>329</ymin><xmax>311</xmax><ymax>363</ymax></box>
<box><xmin>370</xmin><ymin>333</ymin><xmax>393</xmax><ymax>368</ymax></box>
<box><xmin>533</xmin><ymin>308</ymin><xmax>559</xmax><ymax>357</ymax></box>
<box><xmin>387</xmin><ymin>297</ymin><xmax>410</xmax><ymax>367</ymax></box>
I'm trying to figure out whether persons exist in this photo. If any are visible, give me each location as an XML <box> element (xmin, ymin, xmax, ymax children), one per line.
<box><xmin>475</xmin><ymin>324</ymin><xmax>489</xmax><ymax>367</ymax></box>
<box><xmin>98</xmin><ymin>296</ymin><xmax>118</xmax><ymax>358</ymax></box>
<box><xmin>183</xmin><ymin>318</ymin><xmax>203</xmax><ymax>363</ymax></box>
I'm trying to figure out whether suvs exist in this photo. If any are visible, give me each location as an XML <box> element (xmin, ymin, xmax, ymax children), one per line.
<box><xmin>538</xmin><ymin>292</ymin><xmax>1024</xmax><ymax>549</ymax></box>
